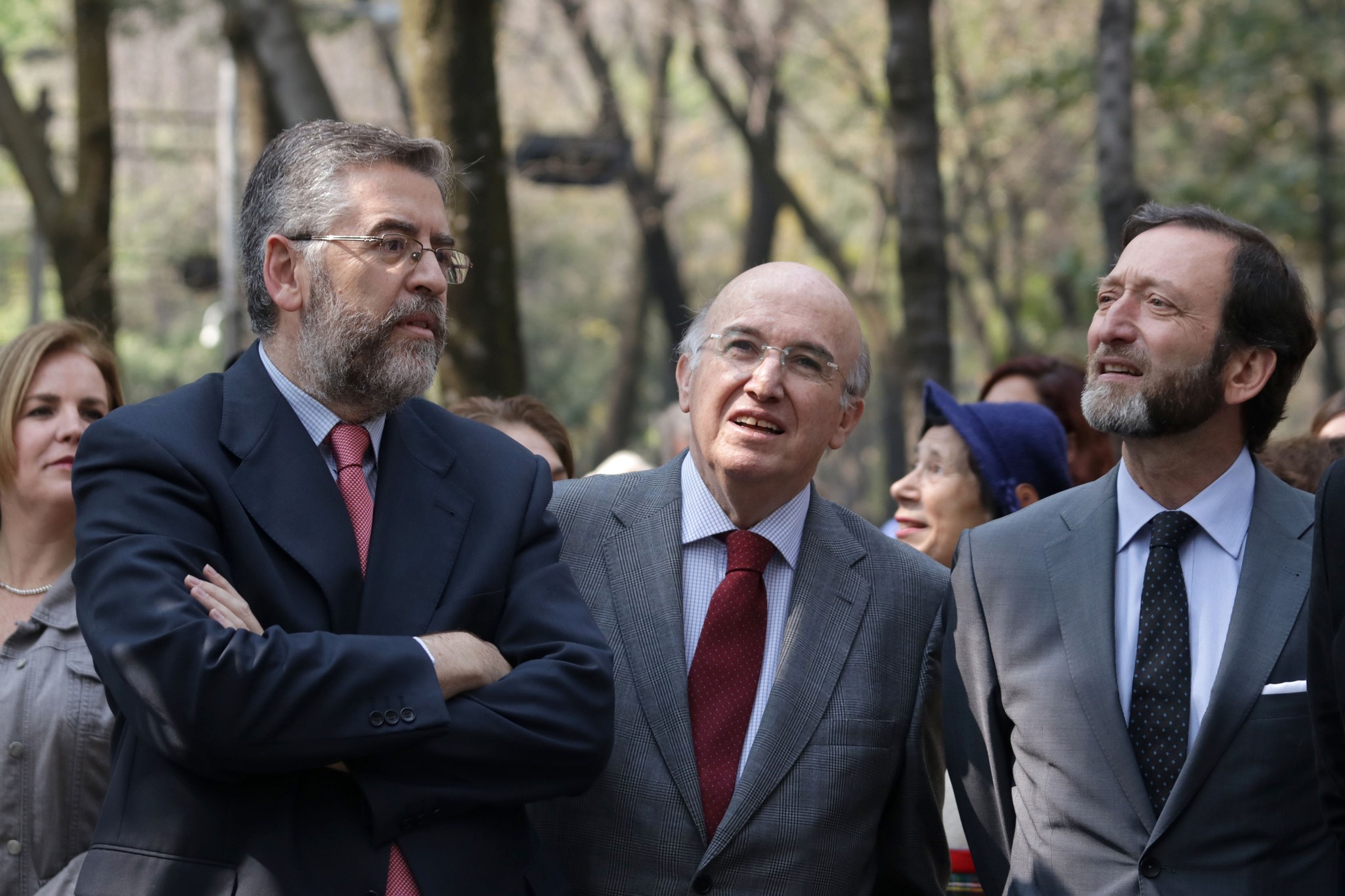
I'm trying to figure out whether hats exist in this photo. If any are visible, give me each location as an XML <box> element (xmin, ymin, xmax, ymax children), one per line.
<box><xmin>918</xmin><ymin>380</ymin><xmax>1074</xmax><ymax>522</ymax></box>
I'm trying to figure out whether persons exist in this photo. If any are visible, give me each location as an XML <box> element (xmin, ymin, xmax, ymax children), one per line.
<box><xmin>0</xmin><ymin>320</ymin><xmax>121</xmax><ymax>894</ymax></box>
<box><xmin>885</xmin><ymin>374</ymin><xmax>1082</xmax><ymax>896</ymax></box>
<box><xmin>77</xmin><ymin>117</ymin><xmax>619</xmax><ymax>896</ymax></box>
<box><xmin>1312</xmin><ymin>392</ymin><xmax>1343</xmax><ymax>441</ymax></box>
<box><xmin>516</xmin><ymin>261</ymin><xmax>961</xmax><ymax>896</ymax></box>
<box><xmin>439</xmin><ymin>394</ymin><xmax>574</xmax><ymax>487</ymax></box>
<box><xmin>974</xmin><ymin>353</ymin><xmax>1120</xmax><ymax>491</ymax></box>
<box><xmin>939</xmin><ymin>196</ymin><xmax>1344</xmax><ymax>896</ymax></box>
<box><xmin>650</xmin><ymin>399</ymin><xmax>689</xmax><ymax>467</ymax></box>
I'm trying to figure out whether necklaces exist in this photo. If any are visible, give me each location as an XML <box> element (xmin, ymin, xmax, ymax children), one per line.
<box><xmin>1</xmin><ymin>578</ymin><xmax>57</xmax><ymax>600</ymax></box>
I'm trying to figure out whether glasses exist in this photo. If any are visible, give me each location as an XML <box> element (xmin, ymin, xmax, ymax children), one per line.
<box><xmin>286</xmin><ymin>233</ymin><xmax>474</xmax><ymax>285</ymax></box>
<box><xmin>696</xmin><ymin>330</ymin><xmax>849</xmax><ymax>393</ymax></box>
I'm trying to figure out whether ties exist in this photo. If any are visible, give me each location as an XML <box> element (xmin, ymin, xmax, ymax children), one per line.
<box><xmin>686</xmin><ymin>531</ymin><xmax>776</xmax><ymax>845</ymax></box>
<box><xmin>1128</xmin><ymin>511</ymin><xmax>1205</xmax><ymax>822</ymax></box>
<box><xmin>326</xmin><ymin>423</ymin><xmax>372</xmax><ymax>579</ymax></box>
